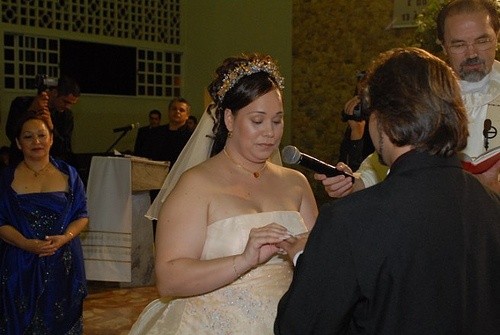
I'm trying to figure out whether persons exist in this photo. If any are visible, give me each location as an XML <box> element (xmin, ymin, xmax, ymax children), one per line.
<box><xmin>133</xmin><ymin>109</ymin><xmax>161</xmax><ymax>153</ymax></box>
<box><xmin>339</xmin><ymin>71</ymin><xmax>376</xmax><ymax>169</ymax></box>
<box><xmin>6</xmin><ymin>75</ymin><xmax>81</xmax><ymax>159</ymax></box>
<box><xmin>0</xmin><ymin>110</ymin><xmax>90</xmax><ymax>335</ymax></box>
<box><xmin>187</xmin><ymin>116</ymin><xmax>197</xmax><ymax>128</ymax></box>
<box><xmin>313</xmin><ymin>0</ymin><xmax>500</xmax><ymax>199</ymax></box>
<box><xmin>274</xmin><ymin>47</ymin><xmax>499</xmax><ymax>335</ymax></box>
<box><xmin>148</xmin><ymin>98</ymin><xmax>192</xmax><ymax>161</ymax></box>
<box><xmin>127</xmin><ymin>51</ymin><xmax>320</xmax><ymax>334</ymax></box>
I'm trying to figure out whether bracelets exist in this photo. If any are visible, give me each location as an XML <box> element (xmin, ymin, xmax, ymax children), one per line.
<box><xmin>67</xmin><ymin>232</ymin><xmax>76</xmax><ymax>241</ymax></box>
<box><xmin>232</xmin><ymin>254</ymin><xmax>244</xmax><ymax>280</ymax></box>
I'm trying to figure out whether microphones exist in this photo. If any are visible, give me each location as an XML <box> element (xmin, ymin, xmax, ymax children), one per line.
<box><xmin>483</xmin><ymin>119</ymin><xmax>491</xmax><ymax>132</ymax></box>
<box><xmin>113</xmin><ymin>122</ymin><xmax>141</xmax><ymax>133</ymax></box>
<box><xmin>282</xmin><ymin>145</ymin><xmax>355</xmax><ymax>184</ymax></box>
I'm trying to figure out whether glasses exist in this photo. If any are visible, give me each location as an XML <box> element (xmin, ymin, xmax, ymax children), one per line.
<box><xmin>443</xmin><ymin>38</ymin><xmax>497</xmax><ymax>51</ymax></box>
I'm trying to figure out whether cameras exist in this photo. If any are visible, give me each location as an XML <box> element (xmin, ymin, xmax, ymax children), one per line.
<box><xmin>35</xmin><ymin>74</ymin><xmax>57</xmax><ymax>90</ymax></box>
<box><xmin>353</xmin><ymin>94</ymin><xmax>362</xmax><ymax>119</ymax></box>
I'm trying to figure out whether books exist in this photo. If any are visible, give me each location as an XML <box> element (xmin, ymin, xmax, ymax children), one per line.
<box><xmin>459</xmin><ymin>145</ymin><xmax>500</xmax><ymax>176</ymax></box>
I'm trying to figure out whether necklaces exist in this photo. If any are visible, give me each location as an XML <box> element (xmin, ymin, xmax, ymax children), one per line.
<box><xmin>222</xmin><ymin>145</ymin><xmax>268</xmax><ymax>179</ymax></box>
<box><xmin>23</xmin><ymin>158</ymin><xmax>52</xmax><ymax>177</ymax></box>
